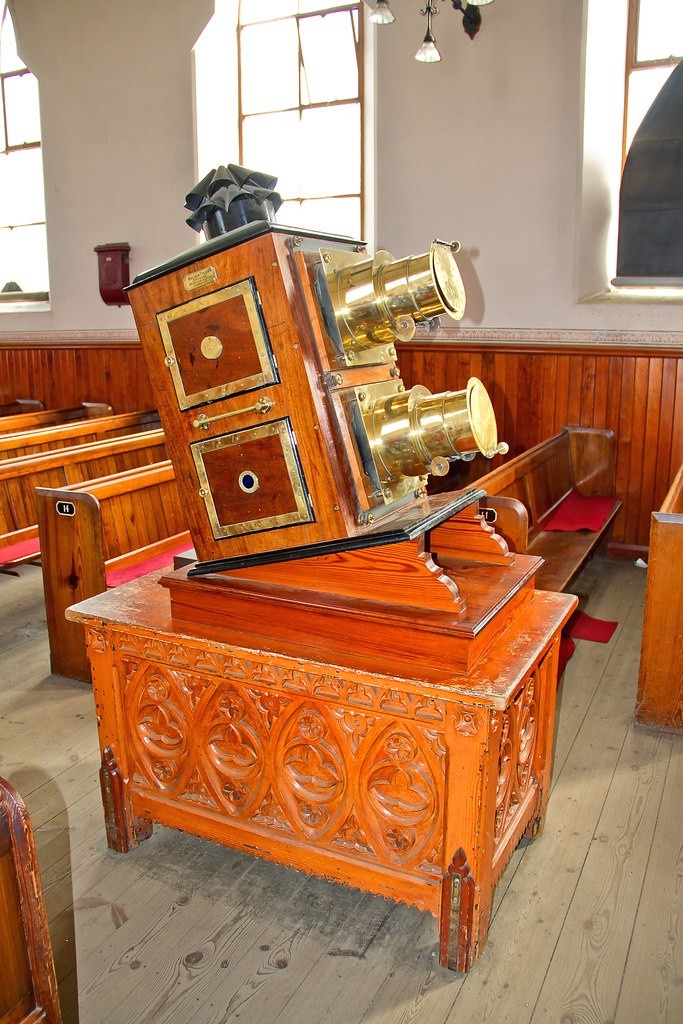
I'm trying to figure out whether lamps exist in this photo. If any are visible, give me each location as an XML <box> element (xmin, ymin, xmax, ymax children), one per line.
<box><xmin>369</xmin><ymin>1</ymin><xmax>495</xmax><ymax>64</ymax></box>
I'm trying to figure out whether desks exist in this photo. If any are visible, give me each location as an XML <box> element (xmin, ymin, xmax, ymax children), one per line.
<box><xmin>66</xmin><ymin>562</ymin><xmax>582</xmax><ymax>973</ymax></box>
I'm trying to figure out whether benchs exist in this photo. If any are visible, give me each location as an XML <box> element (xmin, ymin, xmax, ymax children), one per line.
<box><xmin>1</xmin><ymin>400</ymin><xmax>682</xmax><ymax>741</ymax></box>
